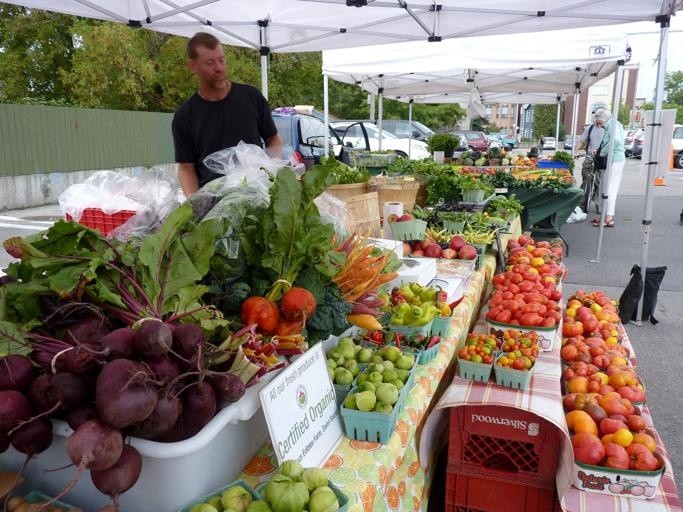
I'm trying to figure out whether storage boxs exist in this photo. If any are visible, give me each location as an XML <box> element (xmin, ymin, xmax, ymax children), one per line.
<box><xmin>1</xmin><ymin>354</ymin><xmax>289</xmax><ymax>512</ymax></box>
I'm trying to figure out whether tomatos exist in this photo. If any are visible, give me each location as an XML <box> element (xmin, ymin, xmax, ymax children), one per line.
<box><xmin>561</xmin><ymin>334</ymin><xmax>642</xmax><ymax>401</ymax></box>
<box><xmin>563</xmin><ymin>289</ymin><xmax>618</xmax><ymax>338</ymax></box>
<box><xmin>506</xmin><ymin>236</ymin><xmax>564</xmax><ymax>265</ymax></box>
<box><xmin>387</xmin><ymin>214</ymin><xmax>397</xmax><ymax>222</ymax></box>
<box><xmin>564</xmin><ymin>392</ymin><xmax>656</xmax><ymax>472</ymax></box>
<box><xmin>400</xmin><ymin>236</ymin><xmax>477</xmax><ymax>259</ymax></box>
<box><xmin>397</xmin><ymin>214</ymin><xmax>411</xmax><ymax>222</ymax></box>
<box><xmin>461</xmin><ymin>328</ymin><xmax>541</xmax><ymax>374</ymax></box>
<box><xmin>487</xmin><ymin>263</ymin><xmax>561</xmax><ymax>328</ymax></box>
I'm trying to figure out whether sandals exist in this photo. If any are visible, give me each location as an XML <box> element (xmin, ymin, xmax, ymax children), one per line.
<box><xmin>593</xmin><ymin>219</ymin><xmax>613</xmax><ymax>226</ymax></box>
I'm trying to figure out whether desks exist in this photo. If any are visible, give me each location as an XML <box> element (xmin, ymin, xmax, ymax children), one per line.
<box><xmin>242</xmin><ymin>215</ymin><xmax>524</xmax><ymax>512</ymax></box>
<box><xmin>507</xmin><ymin>188</ymin><xmax>586</xmax><ymax>257</ymax></box>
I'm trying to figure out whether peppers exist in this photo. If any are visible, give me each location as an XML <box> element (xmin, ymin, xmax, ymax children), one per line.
<box><xmin>377</xmin><ymin>278</ymin><xmax>467</xmax><ymax>323</ymax></box>
<box><xmin>367</xmin><ymin>328</ymin><xmax>442</xmax><ymax>350</ymax></box>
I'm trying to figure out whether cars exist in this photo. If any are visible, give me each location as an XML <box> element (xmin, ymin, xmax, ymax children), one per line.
<box><xmin>617</xmin><ymin>122</ymin><xmax>682</xmax><ymax>171</ymax></box>
<box><xmin>562</xmin><ymin>137</ymin><xmax>580</xmax><ymax>151</ymax></box>
<box><xmin>375</xmin><ymin>115</ymin><xmax>442</xmax><ymax>158</ymax></box>
<box><xmin>536</xmin><ymin>133</ymin><xmax>558</xmax><ymax>152</ymax></box>
<box><xmin>444</xmin><ymin>129</ymin><xmax>489</xmax><ymax>153</ymax></box>
<box><xmin>263</xmin><ymin>109</ymin><xmax>368</xmax><ymax>174</ymax></box>
<box><xmin>329</xmin><ymin>119</ymin><xmax>437</xmax><ymax>170</ymax></box>
<box><xmin>484</xmin><ymin>132</ymin><xmax>521</xmax><ymax>154</ymax></box>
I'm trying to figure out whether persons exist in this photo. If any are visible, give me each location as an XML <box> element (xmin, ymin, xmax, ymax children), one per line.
<box><xmin>574</xmin><ymin>106</ymin><xmax>609</xmax><ymax>216</ymax></box>
<box><xmin>171</xmin><ymin>33</ymin><xmax>281</xmax><ymax>200</ymax></box>
<box><xmin>592</xmin><ymin>108</ymin><xmax>625</xmax><ymax>228</ymax></box>
<box><xmin>535</xmin><ymin>133</ymin><xmax>546</xmax><ymax>157</ymax></box>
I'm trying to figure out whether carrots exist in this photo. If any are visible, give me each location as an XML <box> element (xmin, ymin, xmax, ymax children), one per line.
<box><xmin>329</xmin><ymin>225</ymin><xmax>398</xmax><ymax>328</ymax></box>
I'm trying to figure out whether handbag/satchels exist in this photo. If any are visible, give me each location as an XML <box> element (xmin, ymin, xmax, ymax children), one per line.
<box><xmin>593</xmin><ymin>155</ymin><xmax>607</xmax><ymax>168</ymax></box>
<box><xmin>618</xmin><ymin>264</ymin><xmax>667</xmax><ymax>324</ymax></box>
